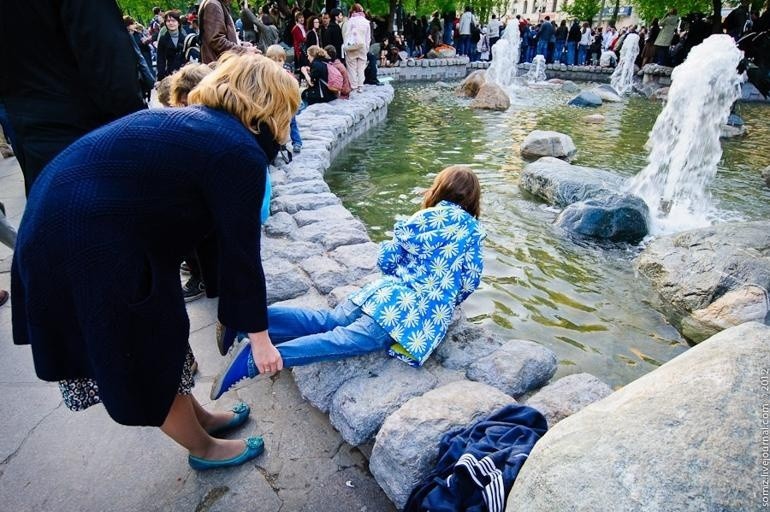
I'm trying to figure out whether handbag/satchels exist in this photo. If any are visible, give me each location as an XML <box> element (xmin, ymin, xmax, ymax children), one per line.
<box><xmin>471</xmin><ymin>14</ymin><xmax>476</xmax><ymax>32</ymax></box>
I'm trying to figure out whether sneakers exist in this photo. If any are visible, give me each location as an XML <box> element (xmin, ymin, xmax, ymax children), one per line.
<box><xmin>215</xmin><ymin>319</ymin><xmax>236</xmax><ymax>357</ymax></box>
<box><xmin>180</xmin><ymin>262</ymin><xmax>191</xmax><ymax>274</ymax></box>
<box><xmin>182</xmin><ymin>279</ymin><xmax>206</xmax><ymax>303</ymax></box>
<box><xmin>210</xmin><ymin>337</ymin><xmax>260</xmax><ymax>400</ymax></box>
<box><xmin>293</xmin><ymin>143</ymin><xmax>303</xmax><ymax>152</ymax></box>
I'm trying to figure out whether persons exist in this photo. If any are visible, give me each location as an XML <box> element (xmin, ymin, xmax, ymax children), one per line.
<box><xmin>240</xmin><ymin>2</ymin><xmax>256</xmax><ymax>44</ymax></box>
<box><xmin>554</xmin><ymin>20</ymin><xmax>568</xmax><ymax>64</ymax></box>
<box><xmin>150</xmin><ymin>7</ymin><xmax>167</xmax><ymax>39</ymax></box>
<box><xmin>388</xmin><ymin>47</ymin><xmax>400</xmax><ymax>66</ymax></box>
<box><xmin>0</xmin><ymin>2</ymin><xmax>149</xmax><ymax>214</ymax></box>
<box><xmin>286</xmin><ymin>13</ymin><xmax>308</xmax><ymax>67</ymax></box>
<box><xmin>405</xmin><ymin>16</ymin><xmax>417</xmax><ymax>58</ymax></box>
<box><xmin>456</xmin><ymin>5</ymin><xmax>476</xmax><ymax>56</ymax></box>
<box><xmin>210</xmin><ymin>165</ymin><xmax>484</xmax><ymax>401</ymax></box>
<box><xmin>284</xmin><ymin>7</ymin><xmax>305</xmax><ymax>45</ymax></box>
<box><xmin>245</xmin><ymin>7</ymin><xmax>279</xmax><ymax>49</ymax></box>
<box><xmin>418</xmin><ymin>14</ymin><xmax>429</xmax><ymax>60</ymax></box>
<box><xmin>596</xmin><ymin>24</ymin><xmax>648</xmax><ymax>67</ymax></box>
<box><xmin>316</xmin><ymin>13</ymin><xmax>342</xmax><ymax>53</ymax></box>
<box><xmin>341</xmin><ymin>3</ymin><xmax>371</xmax><ymax>94</ymax></box>
<box><xmin>269</xmin><ymin>5</ymin><xmax>287</xmax><ymax>43</ymax></box>
<box><xmin>430</xmin><ymin>11</ymin><xmax>441</xmax><ymax>49</ymax></box>
<box><xmin>485</xmin><ymin>13</ymin><xmax>503</xmax><ymax>60</ymax></box>
<box><xmin>471</xmin><ymin>31</ymin><xmax>484</xmax><ymax>62</ymax></box>
<box><xmin>258</xmin><ymin>43</ymin><xmax>301</xmax><ymax>155</ymax></box>
<box><xmin>523</xmin><ymin>27</ymin><xmax>533</xmax><ymax>63</ymax></box>
<box><xmin>442</xmin><ymin>8</ymin><xmax>453</xmax><ymax>46</ymax></box>
<box><xmin>324</xmin><ymin>45</ymin><xmax>351</xmax><ymax>99</ymax></box>
<box><xmin>305</xmin><ymin>16</ymin><xmax>322</xmax><ymax>62</ymax></box>
<box><xmin>580</xmin><ymin>22</ymin><xmax>594</xmax><ymax>65</ymax></box>
<box><xmin>198</xmin><ymin>0</ymin><xmax>250</xmax><ymax>63</ymax></box>
<box><xmin>12</xmin><ymin>52</ymin><xmax>300</xmax><ymax>470</ymax></box>
<box><xmin>569</xmin><ymin>18</ymin><xmax>580</xmax><ymax>64</ymax></box>
<box><xmin>155</xmin><ymin>12</ymin><xmax>193</xmax><ymax>80</ymax></box>
<box><xmin>654</xmin><ymin>9</ymin><xmax>679</xmax><ymax>65</ymax></box>
<box><xmin>380</xmin><ymin>38</ymin><xmax>392</xmax><ymax>66</ymax></box>
<box><xmin>298</xmin><ymin>44</ymin><xmax>342</xmax><ymax>105</ymax></box>
<box><xmin>517</xmin><ymin>14</ymin><xmax>528</xmax><ymax>36</ymax></box>
<box><xmin>480</xmin><ymin>28</ymin><xmax>492</xmax><ymax>60</ymax></box>
<box><xmin>330</xmin><ymin>9</ymin><xmax>343</xmax><ymax>52</ymax></box>
<box><xmin>534</xmin><ymin>16</ymin><xmax>553</xmax><ymax>61</ymax></box>
<box><xmin>642</xmin><ymin>19</ymin><xmax>661</xmax><ymax>67</ymax></box>
<box><xmin>122</xmin><ymin>16</ymin><xmax>156</xmax><ymax>95</ymax></box>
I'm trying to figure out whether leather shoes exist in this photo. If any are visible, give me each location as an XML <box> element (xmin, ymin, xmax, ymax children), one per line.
<box><xmin>207</xmin><ymin>402</ymin><xmax>250</xmax><ymax>435</ymax></box>
<box><xmin>188</xmin><ymin>436</ymin><xmax>264</xmax><ymax>471</ymax></box>
<box><xmin>0</xmin><ymin>290</ymin><xmax>10</xmax><ymax>304</ymax></box>
